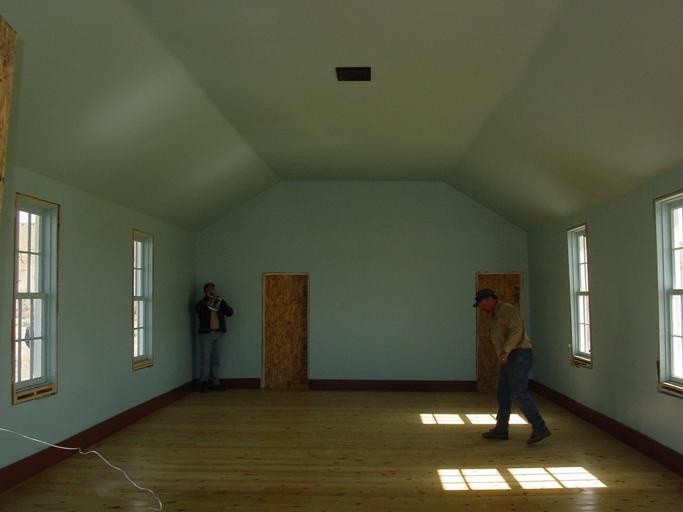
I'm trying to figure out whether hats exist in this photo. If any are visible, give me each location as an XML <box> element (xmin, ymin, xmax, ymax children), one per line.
<box><xmin>473</xmin><ymin>288</ymin><xmax>496</xmax><ymax>307</ymax></box>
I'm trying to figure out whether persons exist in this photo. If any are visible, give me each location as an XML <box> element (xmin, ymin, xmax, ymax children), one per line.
<box><xmin>193</xmin><ymin>283</ymin><xmax>233</xmax><ymax>393</ymax></box>
<box><xmin>472</xmin><ymin>288</ymin><xmax>551</xmax><ymax>444</ymax></box>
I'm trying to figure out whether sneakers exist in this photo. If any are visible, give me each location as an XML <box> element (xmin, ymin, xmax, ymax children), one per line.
<box><xmin>482</xmin><ymin>429</ymin><xmax>509</xmax><ymax>440</ymax></box>
<box><xmin>527</xmin><ymin>425</ymin><xmax>551</xmax><ymax>445</ymax></box>
<box><xmin>199</xmin><ymin>382</ymin><xmax>226</xmax><ymax>393</ymax></box>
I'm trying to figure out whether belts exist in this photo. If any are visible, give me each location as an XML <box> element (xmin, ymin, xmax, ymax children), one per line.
<box><xmin>210</xmin><ymin>329</ymin><xmax>221</xmax><ymax>331</ymax></box>
